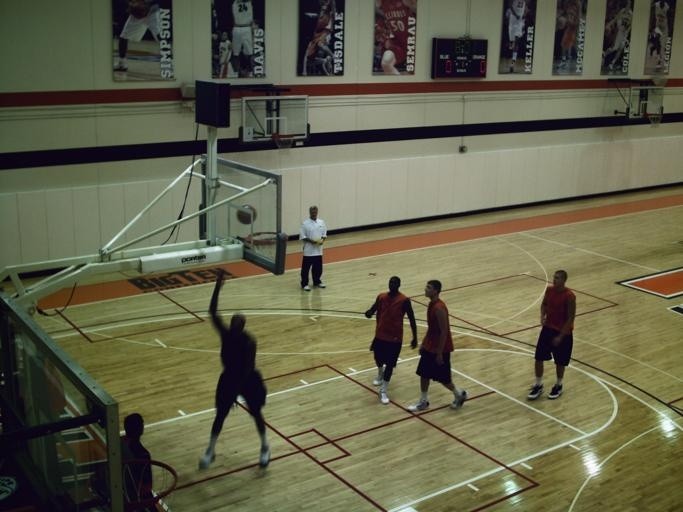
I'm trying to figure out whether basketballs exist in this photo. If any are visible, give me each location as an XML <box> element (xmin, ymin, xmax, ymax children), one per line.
<box><xmin>237</xmin><ymin>205</ymin><xmax>256</xmax><ymax>224</ymax></box>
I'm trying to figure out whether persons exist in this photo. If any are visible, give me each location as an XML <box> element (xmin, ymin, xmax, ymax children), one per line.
<box><xmin>374</xmin><ymin>0</ymin><xmax>416</xmax><ymax>75</ymax></box>
<box><xmin>198</xmin><ymin>271</ymin><xmax>272</xmax><ymax>466</ymax></box>
<box><xmin>365</xmin><ymin>276</ymin><xmax>418</xmax><ymax>404</ymax></box>
<box><xmin>602</xmin><ymin>7</ymin><xmax>633</xmax><ymax>69</ymax></box>
<box><xmin>299</xmin><ymin>206</ymin><xmax>327</xmax><ymax>291</ymax></box>
<box><xmin>503</xmin><ymin>0</ymin><xmax>533</xmax><ymax>72</ymax></box>
<box><xmin>106</xmin><ymin>413</ymin><xmax>161</xmax><ymax>512</ymax></box>
<box><xmin>211</xmin><ymin>0</ymin><xmax>265</xmax><ymax>78</ymax></box>
<box><xmin>556</xmin><ymin>5</ymin><xmax>578</xmax><ymax>69</ymax></box>
<box><xmin>408</xmin><ymin>279</ymin><xmax>469</xmax><ymax>412</ymax></box>
<box><xmin>302</xmin><ymin>0</ymin><xmax>334</xmax><ymax>75</ymax></box>
<box><xmin>113</xmin><ymin>1</ymin><xmax>171</xmax><ymax>72</ymax></box>
<box><xmin>649</xmin><ymin>0</ymin><xmax>672</xmax><ymax>71</ymax></box>
<box><xmin>526</xmin><ymin>270</ymin><xmax>576</xmax><ymax>400</ymax></box>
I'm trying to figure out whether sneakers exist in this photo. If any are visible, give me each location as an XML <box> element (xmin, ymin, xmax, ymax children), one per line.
<box><xmin>318</xmin><ymin>283</ymin><xmax>325</xmax><ymax>288</ymax></box>
<box><xmin>200</xmin><ymin>447</ymin><xmax>215</xmax><ymax>464</ymax></box>
<box><xmin>451</xmin><ymin>391</ymin><xmax>466</xmax><ymax>409</ymax></box>
<box><xmin>527</xmin><ymin>384</ymin><xmax>544</xmax><ymax>400</ymax></box>
<box><xmin>373</xmin><ymin>369</ymin><xmax>384</xmax><ymax>386</ymax></box>
<box><xmin>303</xmin><ymin>284</ymin><xmax>311</xmax><ymax>291</ymax></box>
<box><xmin>547</xmin><ymin>384</ymin><xmax>563</xmax><ymax>400</ymax></box>
<box><xmin>259</xmin><ymin>445</ymin><xmax>269</xmax><ymax>466</ymax></box>
<box><xmin>408</xmin><ymin>399</ymin><xmax>429</xmax><ymax>412</ymax></box>
<box><xmin>378</xmin><ymin>388</ymin><xmax>390</xmax><ymax>404</ymax></box>
